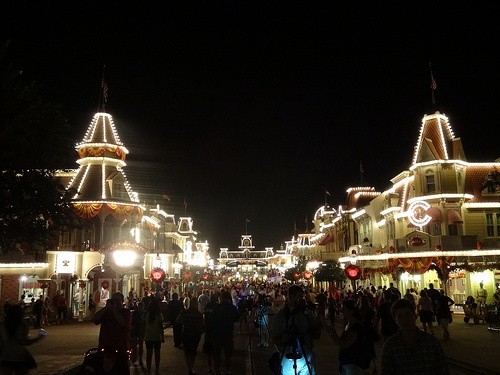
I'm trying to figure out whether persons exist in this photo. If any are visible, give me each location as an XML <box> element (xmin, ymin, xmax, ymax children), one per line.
<box><xmin>0</xmin><ymin>278</ymin><xmax>500</xmax><ymax>375</ymax></box>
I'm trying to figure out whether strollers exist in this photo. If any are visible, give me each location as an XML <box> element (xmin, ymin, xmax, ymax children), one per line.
<box><xmin>79</xmin><ymin>346</ymin><xmax>132</xmax><ymax>375</ymax></box>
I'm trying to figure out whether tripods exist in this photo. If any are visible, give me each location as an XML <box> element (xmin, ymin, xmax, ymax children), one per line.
<box><xmin>275</xmin><ymin>315</ymin><xmax>312</xmax><ymax>375</ymax></box>
<box><xmin>247</xmin><ymin>307</ymin><xmax>276</xmax><ymax>350</ymax></box>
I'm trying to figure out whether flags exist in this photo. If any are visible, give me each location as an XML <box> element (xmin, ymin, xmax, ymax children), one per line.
<box><xmin>102</xmin><ymin>78</ymin><xmax>109</xmax><ymax>103</ymax></box>
<box><xmin>430</xmin><ymin>76</ymin><xmax>437</xmax><ymax>90</ymax></box>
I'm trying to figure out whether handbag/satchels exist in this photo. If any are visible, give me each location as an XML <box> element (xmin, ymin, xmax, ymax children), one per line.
<box><xmin>268</xmin><ymin>351</ymin><xmax>281</xmax><ymax>375</ymax></box>
